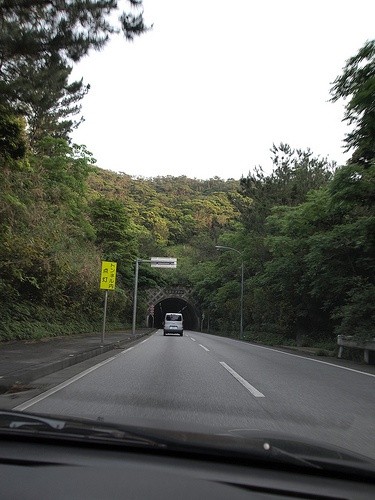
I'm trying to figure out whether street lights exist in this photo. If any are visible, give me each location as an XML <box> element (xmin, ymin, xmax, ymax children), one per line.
<box><xmin>214</xmin><ymin>245</ymin><xmax>244</xmax><ymax>340</ymax></box>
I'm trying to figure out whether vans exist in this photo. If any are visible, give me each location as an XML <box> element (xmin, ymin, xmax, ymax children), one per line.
<box><xmin>163</xmin><ymin>312</ymin><xmax>184</xmax><ymax>336</ymax></box>
